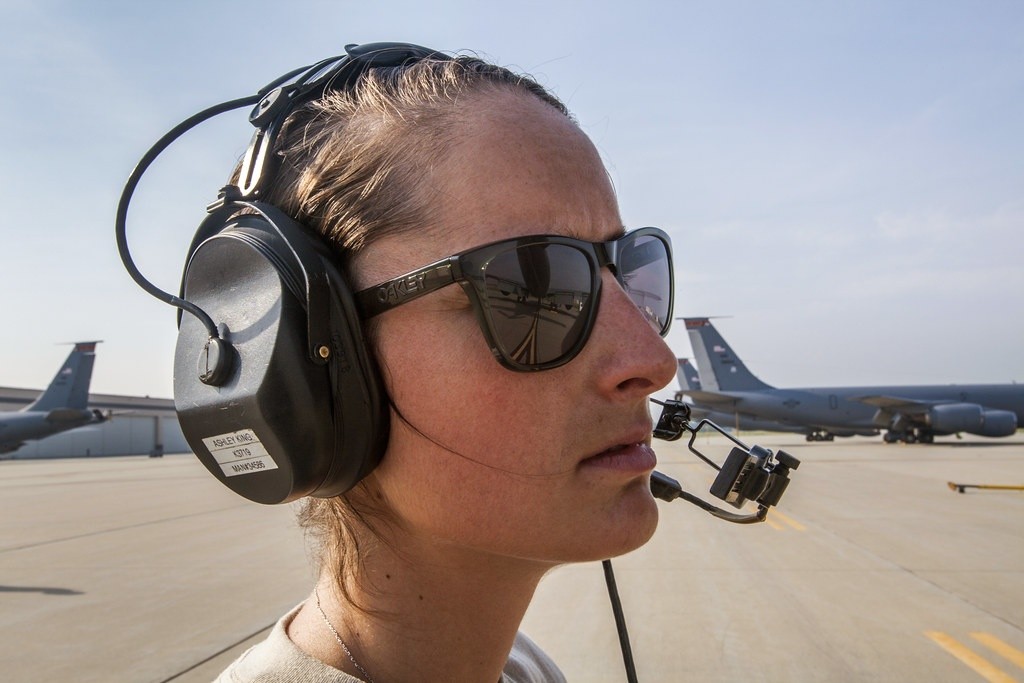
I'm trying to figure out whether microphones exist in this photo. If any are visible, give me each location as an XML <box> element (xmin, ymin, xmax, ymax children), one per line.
<box><xmin>641</xmin><ymin>397</ymin><xmax>800</xmax><ymax>524</ymax></box>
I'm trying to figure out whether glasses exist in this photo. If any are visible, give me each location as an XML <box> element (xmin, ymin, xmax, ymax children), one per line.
<box><xmin>354</xmin><ymin>225</ymin><xmax>675</xmax><ymax>373</ymax></box>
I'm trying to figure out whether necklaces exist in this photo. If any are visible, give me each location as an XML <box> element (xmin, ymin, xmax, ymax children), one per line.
<box><xmin>314</xmin><ymin>585</ymin><xmax>374</xmax><ymax>683</ymax></box>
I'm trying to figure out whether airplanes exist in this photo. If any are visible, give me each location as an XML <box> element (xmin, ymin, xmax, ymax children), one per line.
<box><xmin>672</xmin><ymin>315</ymin><xmax>1024</xmax><ymax>445</ymax></box>
<box><xmin>0</xmin><ymin>340</ymin><xmax>113</xmax><ymax>453</ymax></box>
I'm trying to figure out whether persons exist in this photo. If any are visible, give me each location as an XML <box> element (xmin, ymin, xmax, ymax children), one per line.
<box><xmin>210</xmin><ymin>56</ymin><xmax>679</xmax><ymax>683</ymax></box>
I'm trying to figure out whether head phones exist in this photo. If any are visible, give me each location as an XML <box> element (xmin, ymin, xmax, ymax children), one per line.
<box><xmin>113</xmin><ymin>44</ymin><xmax>450</xmax><ymax>507</ymax></box>
